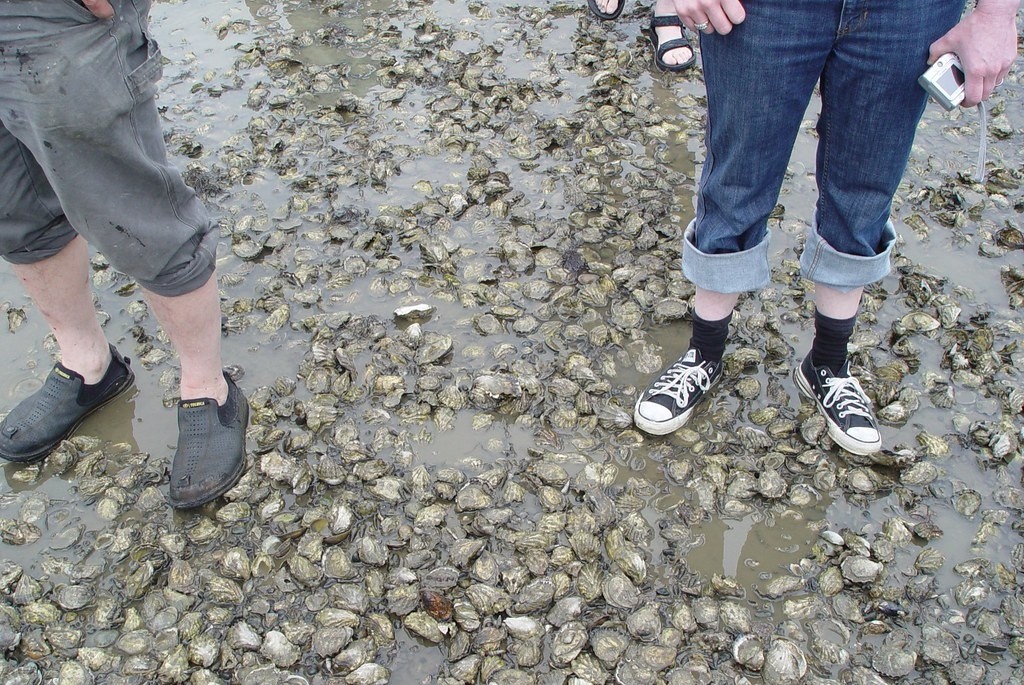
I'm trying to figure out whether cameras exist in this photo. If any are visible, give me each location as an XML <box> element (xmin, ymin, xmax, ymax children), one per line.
<box><xmin>918</xmin><ymin>51</ymin><xmax>966</xmax><ymax>112</ymax></box>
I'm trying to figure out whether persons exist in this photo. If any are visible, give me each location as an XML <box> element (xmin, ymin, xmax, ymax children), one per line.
<box><xmin>587</xmin><ymin>0</ymin><xmax>697</xmax><ymax>72</ymax></box>
<box><xmin>634</xmin><ymin>0</ymin><xmax>1017</xmax><ymax>458</ymax></box>
<box><xmin>0</xmin><ymin>0</ymin><xmax>250</xmax><ymax>509</ymax></box>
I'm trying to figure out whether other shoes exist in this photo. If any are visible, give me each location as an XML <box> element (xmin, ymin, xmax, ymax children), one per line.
<box><xmin>0</xmin><ymin>344</ymin><xmax>135</xmax><ymax>464</ymax></box>
<box><xmin>168</xmin><ymin>370</ymin><xmax>249</xmax><ymax>509</ymax></box>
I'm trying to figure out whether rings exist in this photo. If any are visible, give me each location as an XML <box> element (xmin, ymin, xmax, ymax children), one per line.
<box><xmin>995</xmin><ymin>77</ymin><xmax>1004</xmax><ymax>87</ymax></box>
<box><xmin>694</xmin><ymin>20</ymin><xmax>710</xmax><ymax>31</ymax></box>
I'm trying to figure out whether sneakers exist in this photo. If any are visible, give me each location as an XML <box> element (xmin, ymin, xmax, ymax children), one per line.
<box><xmin>633</xmin><ymin>348</ymin><xmax>723</xmax><ymax>435</ymax></box>
<box><xmin>792</xmin><ymin>350</ymin><xmax>882</xmax><ymax>455</ymax></box>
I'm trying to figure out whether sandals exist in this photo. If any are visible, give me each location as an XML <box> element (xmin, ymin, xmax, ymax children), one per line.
<box><xmin>649</xmin><ymin>10</ymin><xmax>696</xmax><ymax>72</ymax></box>
<box><xmin>587</xmin><ymin>0</ymin><xmax>625</xmax><ymax>19</ymax></box>
